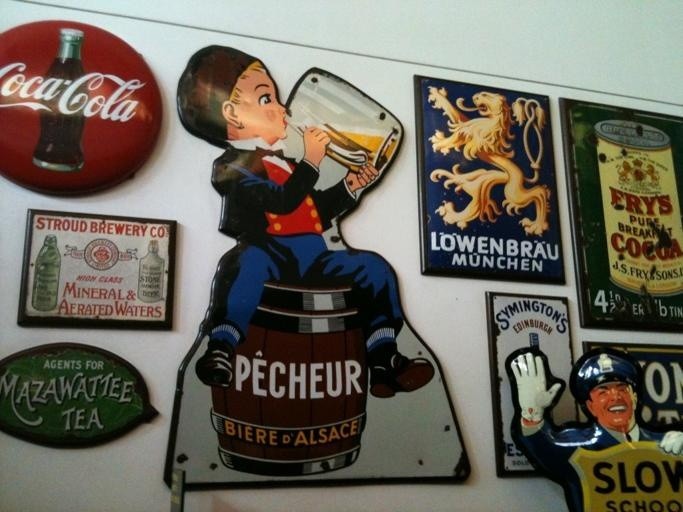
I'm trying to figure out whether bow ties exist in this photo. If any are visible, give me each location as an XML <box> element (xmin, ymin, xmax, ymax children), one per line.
<box><xmin>256</xmin><ymin>145</ymin><xmax>284</xmax><ymax>160</ymax></box>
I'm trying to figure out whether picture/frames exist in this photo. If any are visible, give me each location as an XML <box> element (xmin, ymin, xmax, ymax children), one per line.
<box><xmin>484</xmin><ymin>290</ymin><xmax>581</xmax><ymax>479</ymax></box>
<box><xmin>16</xmin><ymin>207</ymin><xmax>178</xmax><ymax>330</ymax></box>
<box><xmin>560</xmin><ymin>97</ymin><xmax>683</xmax><ymax>333</ymax></box>
<box><xmin>0</xmin><ymin>342</ymin><xmax>159</xmax><ymax>450</ymax></box>
<box><xmin>411</xmin><ymin>73</ymin><xmax>565</xmax><ymax>287</ymax></box>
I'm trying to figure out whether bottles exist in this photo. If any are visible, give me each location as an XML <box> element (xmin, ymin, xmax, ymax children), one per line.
<box><xmin>32</xmin><ymin>232</ymin><xmax>62</xmax><ymax>312</ymax></box>
<box><xmin>139</xmin><ymin>241</ymin><xmax>164</xmax><ymax>303</ymax></box>
<box><xmin>32</xmin><ymin>27</ymin><xmax>86</xmax><ymax>172</ymax></box>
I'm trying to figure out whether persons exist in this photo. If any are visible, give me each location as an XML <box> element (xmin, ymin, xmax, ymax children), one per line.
<box><xmin>177</xmin><ymin>45</ymin><xmax>434</xmax><ymax>398</ymax></box>
<box><xmin>505</xmin><ymin>347</ymin><xmax>683</xmax><ymax>512</ymax></box>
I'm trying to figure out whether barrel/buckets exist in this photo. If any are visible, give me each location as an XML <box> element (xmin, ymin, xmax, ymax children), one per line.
<box><xmin>209</xmin><ymin>280</ymin><xmax>370</xmax><ymax>477</ymax></box>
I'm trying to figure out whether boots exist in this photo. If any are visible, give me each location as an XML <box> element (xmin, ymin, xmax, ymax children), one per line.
<box><xmin>195</xmin><ymin>340</ymin><xmax>235</xmax><ymax>386</ymax></box>
<box><xmin>369</xmin><ymin>342</ymin><xmax>435</xmax><ymax>398</ymax></box>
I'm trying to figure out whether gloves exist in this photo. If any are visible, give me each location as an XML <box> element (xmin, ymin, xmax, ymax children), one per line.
<box><xmin>660</xmin><ymin>430</ymin><xmax>683</xmax><ymax>455</ymax></box>
<box><xmin>511</xmin><ymin>352</ymin><xmax>561</xmax><ymax>421</ymax></box>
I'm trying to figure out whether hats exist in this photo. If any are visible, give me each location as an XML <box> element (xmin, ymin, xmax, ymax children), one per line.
<box><xmin>569</xmin><ymin>349</ymin><xmax>644</xmax><ymax>403</ymax></box>
<box><xmin>177</xmin><ymin>45</ymin><xmax>258</xmax><ymax>149</ymax></box>
